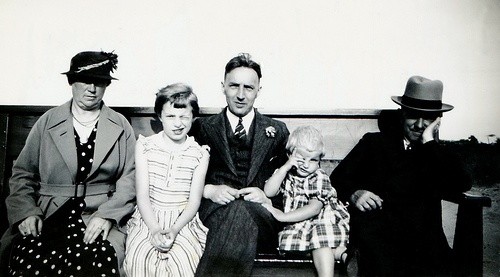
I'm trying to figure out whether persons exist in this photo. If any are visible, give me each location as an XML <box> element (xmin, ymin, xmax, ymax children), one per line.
<box><xmin>329</xmin><ymin>75</ymin><xmax>474</xmax><ymax>277</ymax></box>
<box><xmin>261</xmin><ymin>125</ymin><xmax>360</xmax><ymax>277</ymax></box>
<box><xmin>2</xmin><ymin>51</ymin><xmax>138</xmax><ymax>277</ymax></box>
<box><xmin>185</xmin><ymin>53</ymin><xmax>296</xmax><ymax>277</ymax></box>
<box><xmin>121</xmin><ymin>83</ymin><xmax>212</xmax><ymax>277</ymax></box>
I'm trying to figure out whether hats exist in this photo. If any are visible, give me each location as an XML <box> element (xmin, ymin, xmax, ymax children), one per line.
<box><xmin>61</xmin><ymin>52</ymin><xmax>119</xmax><ymax>85</ymax></box>
<box><xmin>391</xmin><ymin>76</ymin><xmax>454</xmax><ymax>111</ymax></box>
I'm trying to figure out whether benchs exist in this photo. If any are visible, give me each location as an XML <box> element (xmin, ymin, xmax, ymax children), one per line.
<box><xmin>254</xmin><ymin>160</ymin><xmax>491</xmax><ymax>277</ymax></box>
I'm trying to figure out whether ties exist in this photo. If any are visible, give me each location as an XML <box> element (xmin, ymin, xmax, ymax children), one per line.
<box><xmin>235</xmin><ymin>117</ymin><xmax>246</xmax><ymax>138</ymax></box>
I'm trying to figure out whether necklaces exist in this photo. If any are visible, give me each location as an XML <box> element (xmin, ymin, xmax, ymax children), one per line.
<box><xmin>72</xmin><ymin>113</ymin><xmax>101</xmax><ymax>127</ymax></box>
